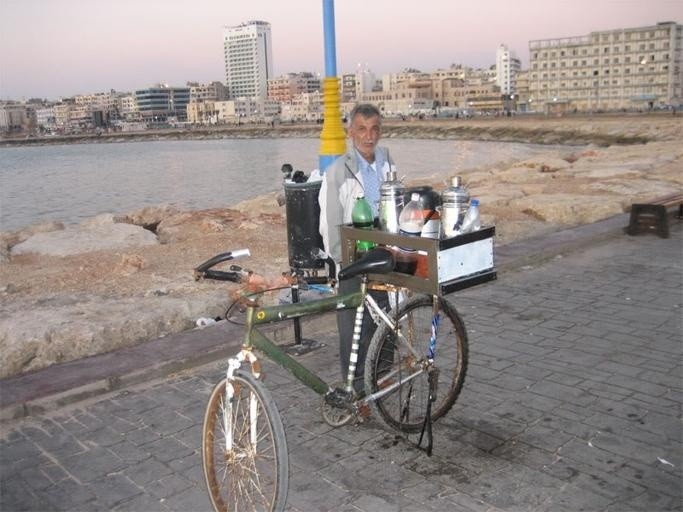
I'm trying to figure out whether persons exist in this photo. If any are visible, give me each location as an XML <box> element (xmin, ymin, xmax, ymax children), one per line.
<box><xmin>316</xmin><ymin>102</ymin><xmax>402</xmax><ymax>425</ymax></box>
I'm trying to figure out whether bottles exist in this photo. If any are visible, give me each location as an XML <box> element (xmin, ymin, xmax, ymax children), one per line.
<box><xmin>377</xmin><ymin>171</ymin><xmax>399</xmax><ymax>250</ymax></box>
<box><xmin>414</xmin><ymin>202</ymin><xmax>439</xmax><ymax>280</ymax></box>
<box><xmin>395</xmin><ymin>192</ymin><xmax>422</xmax><ymax>276</ymax></box>
<box><xmin>441</xmin><ymin>176</ymin><xmax>469</xmax><ymax>241</ymax></box>
<box><xmin>460</xmin><ymin>199</ymin><xmax>482</xmax><ymax>234</ymax></box>
<box><xmin>351</xmin><ymin>192</ymin><xmax>374</xmax><ymax>258</ymax></box>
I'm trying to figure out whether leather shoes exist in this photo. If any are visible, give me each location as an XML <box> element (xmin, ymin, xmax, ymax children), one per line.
<box><xmin>380</xmin><ymin>377</ymin><xmax>396</xmax><ymax>389</ymax></box>
<box><xmin>356</xmin><ymin>402</ymin><xmax>374</xmax><ymax>417</ymax></box>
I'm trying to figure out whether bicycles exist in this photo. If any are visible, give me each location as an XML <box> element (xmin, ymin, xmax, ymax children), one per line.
<box><xmin>191</xmin><ymin>247</ymin><xmax>469</xmax><ymax>512</ymax></box>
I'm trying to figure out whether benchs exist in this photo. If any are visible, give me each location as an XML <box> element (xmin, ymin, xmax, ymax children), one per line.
<box><xmin>627</xmin><ymin>194</ymin><xmax>682</xmax><ymax>239</ymax></box>
